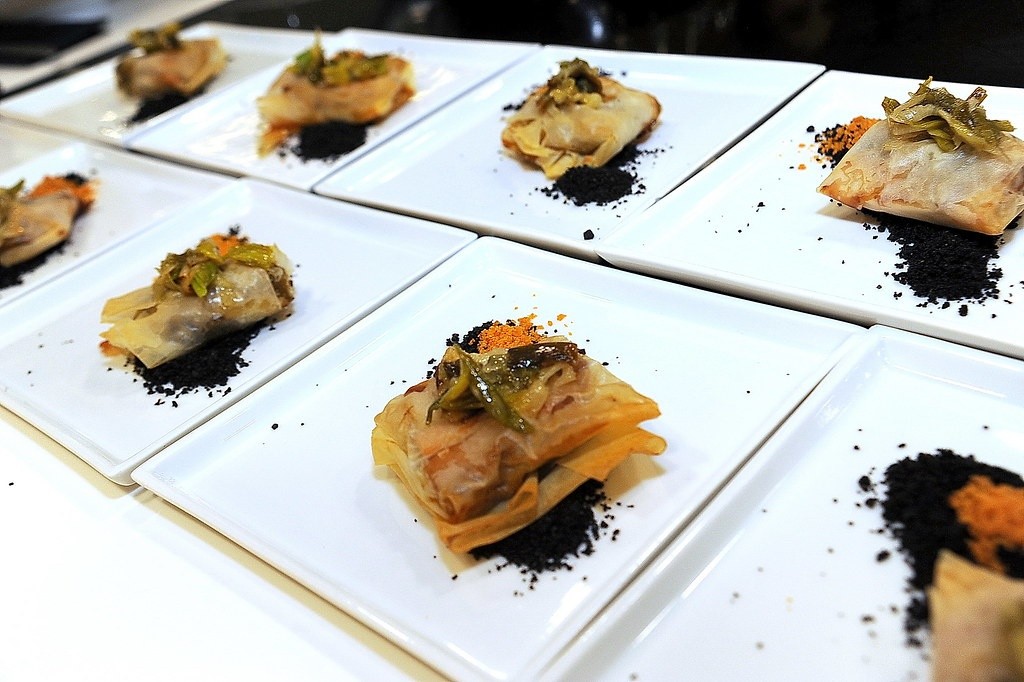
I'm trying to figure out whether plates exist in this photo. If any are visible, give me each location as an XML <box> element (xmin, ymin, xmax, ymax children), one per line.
<box><xmin>0</xmin><ymin>1</ymin><xmax>1023</xmax><ymax>682</ymax></box>
